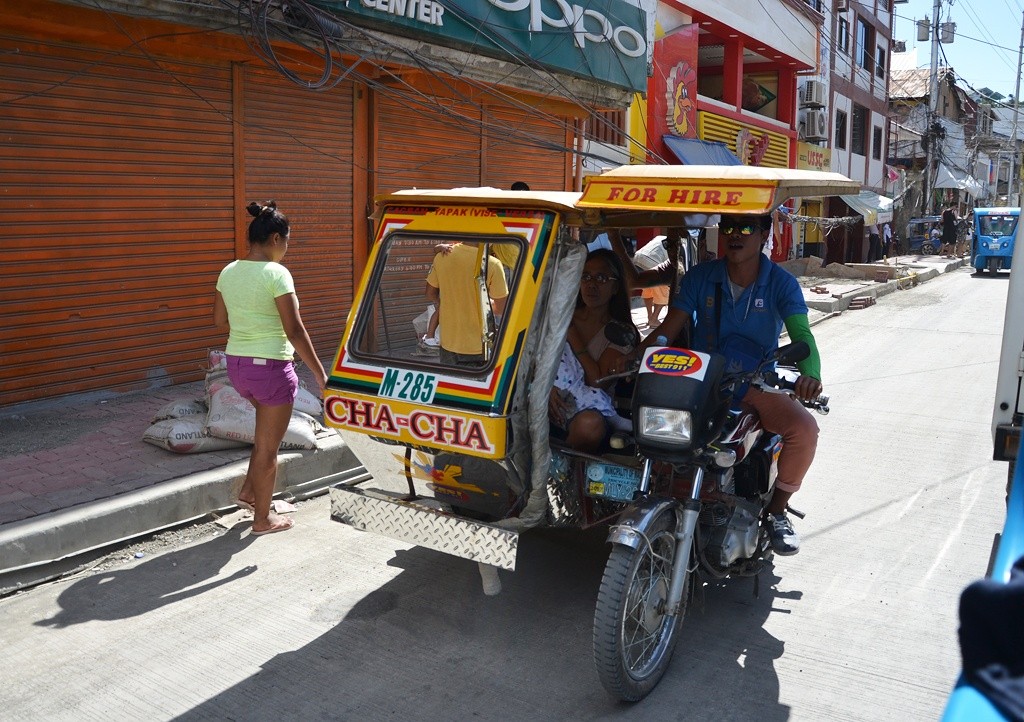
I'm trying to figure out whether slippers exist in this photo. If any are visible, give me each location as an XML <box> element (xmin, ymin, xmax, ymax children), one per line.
<box><xmin>250</xmin><ymin>515</ymin><xmax>294</xmax><ymax>536</ymax></box>
<box><xmin>236</xmin><ymin>499</ymin><xmax>256</xmax><ymax>511</ymax></box>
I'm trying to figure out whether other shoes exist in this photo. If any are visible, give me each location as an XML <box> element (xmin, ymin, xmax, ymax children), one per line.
<box><xmin>421</xmin><ymin>334</ymin><xmax>440</xmax><ymax>347</ymax></box>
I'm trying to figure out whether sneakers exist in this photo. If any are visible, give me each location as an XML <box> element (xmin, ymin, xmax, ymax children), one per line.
<box><xmin>762</xmin><ymin>507</ymin><xmax>800</xmax><ymax>556</ymax></box>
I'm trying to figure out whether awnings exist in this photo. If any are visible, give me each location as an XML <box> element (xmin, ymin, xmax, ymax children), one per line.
<box><xmin>662</xmin><ymin>135</ymin><xmax>742</xmax><ymax>165</ymax></box>
<box><xmin>840</xmin><ymin>190</ymin><xmax>893</xmax><ymax>226</ymax></box>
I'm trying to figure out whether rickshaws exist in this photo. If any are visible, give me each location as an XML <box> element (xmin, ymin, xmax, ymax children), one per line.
<box><xmin>908</xmin><ymin>215</ymin><xmax>973</xmax><ymax>256</ymax></box>
<box><xmin>320</xmin><ymin>164</ymin><xmax>863</xmax><ymax>706</ymax></box>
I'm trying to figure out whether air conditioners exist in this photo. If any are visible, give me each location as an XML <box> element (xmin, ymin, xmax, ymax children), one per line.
<box><xmin>804</xmin><ymin>80</ymin><xmax>826</xmax><ymax>106</ymax></box>
<box><xmin>805</xmin><ymin>110</ymin><xmax>828</xmax><ymax>139</ymax></box>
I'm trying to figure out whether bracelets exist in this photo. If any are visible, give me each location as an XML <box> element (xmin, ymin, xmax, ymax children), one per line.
<box><xmin>574</xmin><ymin>350</ymin><xmax>588</xmax><ymax>355</ymax></box>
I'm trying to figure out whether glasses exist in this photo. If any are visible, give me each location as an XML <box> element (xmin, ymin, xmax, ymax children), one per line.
<box><xmin>718</xmin><ymin>221</ymin><xmax>764</xmax><ymax>236</ymax></box>
<box><xmin>581</xmin><ymin>272</ymin><xmax>618</xmax><ymax>284</ymax></box>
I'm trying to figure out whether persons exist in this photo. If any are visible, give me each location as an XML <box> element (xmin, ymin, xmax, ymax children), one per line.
<box><xmin>421</xmin><ymin>239</ymin><xmax>509</xmax><ymax>367</ymax></box>
<box><xmin>867</xmin><ymin>225</ymin><xmax>879</xmax><ymax>263</ymax></box>
<box><xmin>939</xmin><ymin>201</ymin><xmax>958</xmax><ymax>259</ymax></box>
<box><xmin>932</xmin><ymin>225</ymin><xmax>940</xmax><ymax>238</ymax></box>
<box><xmin>956</xmin><ymin>210</ymin><xmax>974</xmax><ymax>258</ymax></box>
<box><xmin>606</xmin><ymin>228</ymin><xmax>696</xmax><ymax>308</ymax></box>
<box><xmin>489</xmin><ymin>181</ymin><xmax>531</xmax><ymax>323</ymax></box>
<box><xmin>548</xmin><ymin>248</ymin><xmax>639</xmax><ymax>453</ymax></box>
<box><xmin>552</xmin><ymin>344</ymin><xmax>634</xmax><ymax>431</ymax></box>
<box><xmin>635</xmin><ymin>234</ymin><xmax>669</xmax><ymax>329</ymax></box>
<box><xmin>882</xmin><ymin>223</ymin><xmax>891</xmax><ymax>265</ymax></box>
<box><xmin>985</xmin><ymin>217</ymin><xmax>1012</xmax><ymax>235</ymax></box>
<box><xmin>608</xmin><ymin>213</ymin><xmax>822</xmax><ymax>555</ymax></box>
<box><xmin>212</xmin><ymin>202</ymin><xmax>328</xmax><ymax>535</ymax></box>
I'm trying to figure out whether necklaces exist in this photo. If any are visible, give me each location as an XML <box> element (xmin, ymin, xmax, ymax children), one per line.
<box><xmin>730</xmin><ymin>280</ymin><xmax>754</xmax><ymax>323</ymax></box>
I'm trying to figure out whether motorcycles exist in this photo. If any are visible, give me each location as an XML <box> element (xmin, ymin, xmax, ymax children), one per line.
<box><xmin>970</xmin><ymin>207</ymin><xmax>1022</xmax><ymax>279</ymax></box>
